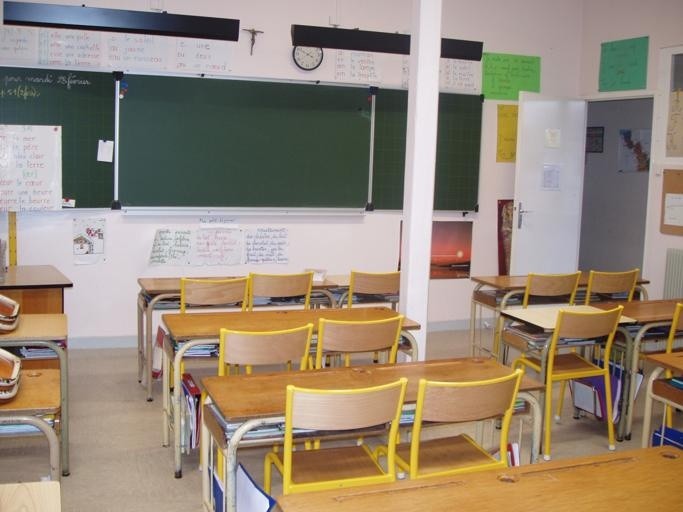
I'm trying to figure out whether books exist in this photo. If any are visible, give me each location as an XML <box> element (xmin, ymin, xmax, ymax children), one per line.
<box><xmin>487</xmin><ymin>442</ymin><xmax>520</xmax><ymax>467</ymax></box>
<box><xmin>568</xmin><ymin>291</ymin><xmax>630</xmax><ymax>303</ymax></box>
<box><xmin>253</xmin><ymin>290</ymin><xmax>329</xmax><ymax>305</ymax></box>
<box><xmin>175</xmin><ymin>340</ymin><xmax>219</xmax><ymax>360</ymax></box>
<box><xmin>18</xmin><ymin>341</ymin><xmax>67</xmax><ymax>359</ymax></box>
<box><xmin>332</xmin><ymin>287</ymin><xmax>361</xmax><ymax>303</ymax></box>
<box><xmin>397</xmin><ymin>334</ymin><xmax>407</xmax><ymax>347</ymax></box>
<box><xmin>667</xmin><ymin>376</ymin><xmax>683</xmax><ymax>390</ymax></box>
<box><xmin>504</xmin><ymin>323</ymin><xmax>600</xmax><ymax>346</ymax></box>
<box><xmin>480</xmin><ymin>289</ymin><xmax>523</xmax><ymax>304</ymax></box>
<box><xmin>0</xmin><ymin>414</ymin><xmax>56</xmax><ymax>435</ymax></box>
<box><xmin>513</xmin><ymin>396</ymin><xmax>526</xmax><ymax>412</ymax></box>
<box><xmin>390</xmin><ymin>398</ymin><xmax>417</xmax><ymax>426</ymax></box>
<box><xmin>142</xmin><ymin>294</ymin><xmax>238</xmax><ymax>309</ymax></box>
<box><xmin>206</xmin><ymin>401</ymin><xmax>320</xmax><ymax>439</ymax></box>
<box><xmin>309</xmin><ymin>334</ymin><xmax>329</xmax><ymax>353</ymax></box>
<box><xmin>621</xmin><ymin>323</ymin><xmax>683</xmax><ymax>337</ymax></box>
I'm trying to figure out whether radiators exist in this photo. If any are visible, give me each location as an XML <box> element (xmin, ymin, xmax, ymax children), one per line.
<box><xmin>663</xmin><ymin>248</ymin><xmax>683</xmax><ymax>300</ymax></box>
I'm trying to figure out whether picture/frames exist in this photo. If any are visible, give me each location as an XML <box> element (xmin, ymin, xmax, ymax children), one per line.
<box><xmin>586</xmin><ymin>126</ymin><xmax>604</xmax><ymax>153</ymax></box>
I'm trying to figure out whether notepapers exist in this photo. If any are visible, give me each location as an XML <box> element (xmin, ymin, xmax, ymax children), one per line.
<box><xmin>97</xmin><ymin>139</ymin><xmax>114</xmax><ymax>162</ymax></box>
<box><xmin>61</xmin><ymin>199</ymin><xmax>76</xmax><ymax>207</ymax></box>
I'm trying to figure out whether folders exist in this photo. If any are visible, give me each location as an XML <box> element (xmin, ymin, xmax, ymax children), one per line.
<box><xmin>182</xmin><ymin>373</ymin><xmax>202</xmax><ymax>448</ymax></box>
<box><xmin>591</xmin><ymin>355</ymin><xmax>644</xmax><ymax>401</ymax></box>
<box><xmin>208</xmin><ymin>462</ymin><xmax>276</xmax><ymax>512</ymax></box>
<box><xmin>652</xmin><ymin>425</ymin><xmax>683</xmax><ymax>450</ymax></box>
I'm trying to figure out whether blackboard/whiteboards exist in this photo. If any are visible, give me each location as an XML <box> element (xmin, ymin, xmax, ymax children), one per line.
<box><xmin>112</xmin><ymin>71</ymin><xmax>379</xmax><ymax>212</ymax></box>
<box><xmin>0</xmin><ymin>66</ymin><xmax>483</xmax><ymax>212</ymax></box>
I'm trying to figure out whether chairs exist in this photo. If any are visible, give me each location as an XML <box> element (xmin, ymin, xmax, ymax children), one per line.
<box><xmin>314</xmin><ymin>315</ymin><xmax>405</xmax><ymax>449</ymax></box>
<box><xmin>170</xmin><ymin>276</ymin><xmax>250</xmax><ymax>392</ymax></box>
<box><xmin>512</xmin><ymin>305</ymin><xmax>624</xmax><ymax>461</ymax></box>
<box><xmin>667</xmin><ymin>302</ymin><xmax>683</xmax><ymax>429</ymax></box>
<box><xmin>264</xmin><ymin>377</ymin><xmax>408</xmax><ymax>495</ymax></box>
<box><xmin>494</xmin><ymin>271</ymin><xmax>581</xmax><ymax>363</ymax></box>
<box><xmin>571</xmin><ymin>267</ymin><xmax>640</xmax><ymax>363</ymax></box>
<box><xmin>199</xmin><ymin>323</ymin><xmax>314</xmax><ymax>469</ymax></box>
<box><xmin>326</xmin><ymin>272</ymin><xmax>401</xmax><ymax>366</ymax></box>
<box><xmin>374</xmin><ymin>368</ymin><xmax>523</xmax><ymax>479</ymax></box>
<box><xmin>245</xmin><ymin>273</ymin><xmax>314</xmax><ymax>375</ymax></box>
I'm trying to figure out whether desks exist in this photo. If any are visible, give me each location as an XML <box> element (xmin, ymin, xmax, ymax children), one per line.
<box><xmin>0</xmin><ymin>315</ymin><xmax>68</xmax><ymax>475</ymax></box>
<box><xmin>200</xmin><ymin>357</ymin><xmax>547</xmax><ymax>512</ymax></box>
<box><xmin>471</xmin><ymin>275</ymin><xmax>650</xmax><ymax>365</ymax></box>
<box><xmin>0</xmin><ymin>265</ymin><xmax>73</xmax><ymax>314</ymax></box>
<box><xmin>0</xmin><ymin>370</ymin><xmax>60</xmax><ymax>482</ymax></box>
<box><xmin>162</xmin><ymin>307</ymin><xmax>422</xmax><ymax>479</ymax></box>
<box><xmin>640</xmin><ymin>353</ymin><xmax>683</xmax><ymax>449</ymax></box>
<box><xmin>495</xmin><ymin>301</ymin><xmax>683</xmax><ymax>454</ymax></box>
<box><xmin>138</xmin><ymin>277</ymin><xmax>401</xmax><ymax>401</ymax></box>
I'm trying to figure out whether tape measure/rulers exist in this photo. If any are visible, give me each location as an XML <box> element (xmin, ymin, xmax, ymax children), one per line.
<box><xmin>8</xmin><ymin>213</ymin><xmax>18</xmax><ymax>267</ymax></box>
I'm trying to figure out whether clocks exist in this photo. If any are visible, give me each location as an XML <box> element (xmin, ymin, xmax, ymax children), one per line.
<box><xmin>293</xmin><ymin>46</ymin><xmax>323</xmax><ymax>71</ymax></box>
